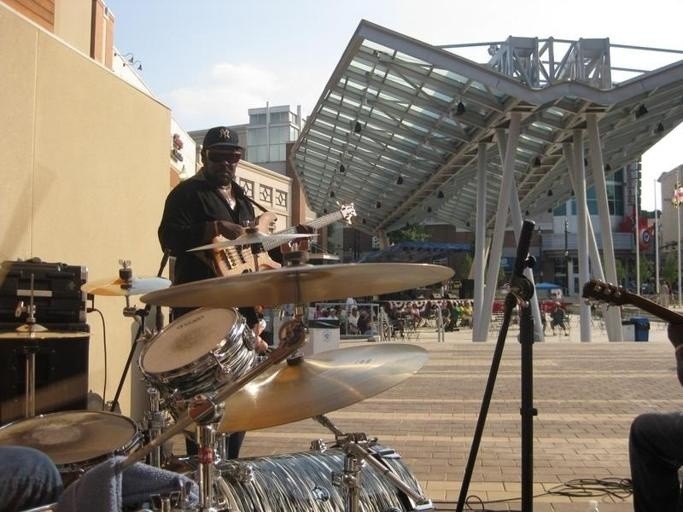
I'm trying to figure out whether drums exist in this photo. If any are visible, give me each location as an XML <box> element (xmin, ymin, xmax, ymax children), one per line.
<box><xmin>136</xmin><ymin>432</ymin><xmax>434</xmax><ymax>512</ymax></box>
<box><xmin>0</xmin><ymin>410</ymin><xmax>145</xmax><ymax>489</ymax></box>
<box><xmin>137</xmin><ymin>306</ymin><xmax>277</xmax><ymax>442</ymax></box>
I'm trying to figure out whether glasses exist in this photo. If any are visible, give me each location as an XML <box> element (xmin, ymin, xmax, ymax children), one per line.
<box><xmin>208</xmin><ymin>151</ymin><xmax>241</xmax><ymax>164</ymax></box>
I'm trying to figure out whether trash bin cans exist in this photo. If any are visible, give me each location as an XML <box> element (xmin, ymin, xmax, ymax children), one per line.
<box><xmin>302</xmin><ymin>319</ymin><xmax>340</xmax><ymax>356</ymax></box>
<box><xmin>622</xmin><ymin>316</ymin><xmax>650</xmax><ymax>342</ymax></box>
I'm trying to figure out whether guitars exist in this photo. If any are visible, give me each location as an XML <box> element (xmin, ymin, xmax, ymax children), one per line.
<box><xmin>581</xmin><ymin>279</ymin><xmax>683</xmax><ymax>326</ymax></box>
<box><xmin>210</xmin><ymin>201</ymin><xmax>358</xmax><ymax>277</ymax></box>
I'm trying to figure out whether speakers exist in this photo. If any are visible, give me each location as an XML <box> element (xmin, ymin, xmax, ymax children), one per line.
<box><xmin>0</xmin><ymin>323</ymin><xmax>89</xmax><ymax>427</ymax></box>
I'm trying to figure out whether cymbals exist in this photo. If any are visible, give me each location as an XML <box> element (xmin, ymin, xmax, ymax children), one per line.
<box><xmin>0</xmin><ymin>324</ymin><xmax>90</xmax><ymax>340</ymax></box>
<box><xmin>81</xmin><ymin>275</ymin><xmax>173</xmax><ymax>296</ymax></box>
<box><xmin>185</xmin><ymin>228</ymin><xmax>319</xmax><ymax>253</ymax></box>
<box><xmin>183</xmin><ymin>344</ymin><xmax>429</xmax><ymax>431</ymax></box>
<box><xmin>140</xmin><ymin>258</ymin><xmax>456</xmax><ymax>307</ymax></box>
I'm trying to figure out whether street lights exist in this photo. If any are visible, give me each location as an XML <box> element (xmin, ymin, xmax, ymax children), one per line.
<box><xmin>672</xmin><ymin>182</ymin><xmax>683</xmax><ymax>306</ymax></box>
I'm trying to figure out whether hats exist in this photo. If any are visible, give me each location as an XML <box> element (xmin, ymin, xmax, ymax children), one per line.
<box><xmin>202</xmin><ymin>127</ymin><xmax>243</xmax><ymax>150</ymax></box>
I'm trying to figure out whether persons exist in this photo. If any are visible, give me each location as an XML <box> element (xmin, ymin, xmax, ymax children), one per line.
<box><xmin>0</xmin><ymin>444</ymin><xmax>63</xmax><ymax>512</ymax></box>
<box><xmin>280</xmin><ymin>302</ymin><xmax>297</xmax><ymax>321</ymax></box>
<box><xmin>549</xmin><ymin>301</ymin><xmax>568</xmax><ymax>336</ymax></box>
<box><xmin>628</xmin><ymin>311</ymin><xmax>682</xmax><ymax>511</ymax></box>
<box><xmin>312</xmin><ymin>299</ymin><xmax>472</xmax><ymax>338</ymax></box>
<box><xmin>254</xmin><ymin>305</ymin><xmax>267</xmax><ymax>338</ymax></box>
<box><xmin>540</xmin><ymin>299</ymin><xmax>554</xmax><ymax>336</ymax></box>
<box><xmin>157</xmin><ymin>122</ymin><xmax>310</xmax><ymax>461</ymax></box>
